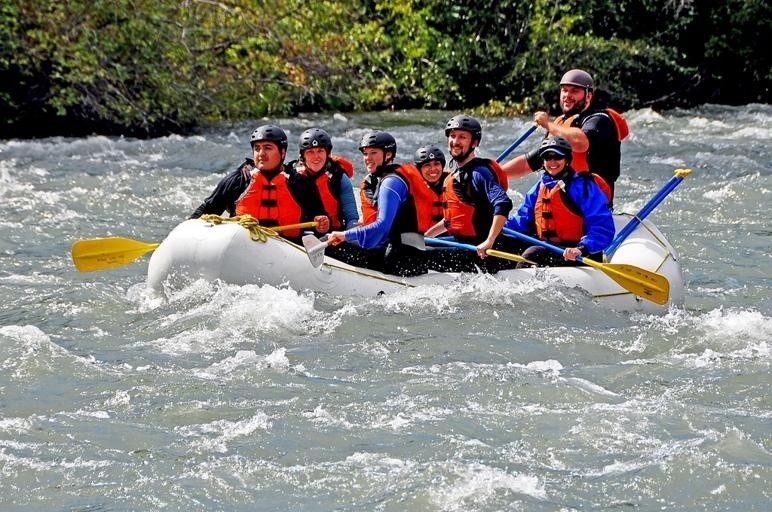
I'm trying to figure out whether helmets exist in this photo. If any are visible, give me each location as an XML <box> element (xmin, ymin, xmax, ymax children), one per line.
<box><xmin>539</xmin><ymin>137</ymin><xmax>572</xmax><ymax>157</ymax></box>
<box><xmin>250</xmin><ymin>114</ymin><xmax>481</xmax><ymax>161</ymax></box>
<box><xmin>560</xmin><ymin>69</ymin><xmax>594</xmax><ymax>88</ymax></box>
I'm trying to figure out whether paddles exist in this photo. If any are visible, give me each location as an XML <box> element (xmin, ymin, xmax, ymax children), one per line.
<box><xmin>72</xmin><ymin>222</ymin><xmax>319</xmax><ymax>271</ymax></box>
<box><xmin>300</xmin><ymin>225</ymin><xmax>363</xmax><ymax>269</ymax></box>
<box><xmin>503</xmin><ymin>227</ymin><xmax>669</xmax><ymax>304</ymax></box>
<box><xmin>424</xmin><ymin>237</ymin><xmax>535</xmax><ymax>263</ymax></box>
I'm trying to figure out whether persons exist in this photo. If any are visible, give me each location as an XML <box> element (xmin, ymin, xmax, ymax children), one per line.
<box><xmin>500</xmin><ymin>68</ymin><xmax>629</xmax><ymax>216</ymax></box>
<box><xmin>285</xmin><ymin>127</ymin><xmax>359</xmax><ymax>239</ymax></box>
<box><xmin>186</xmin><ymin>124</ymin><xmax>332</xmax><ymax>247</ymax></box>
<box><xmin>414</xmin><ymin>143</ymin><xmax>452</xmax><ymax>248</ymax></box>
<box><xmin>497</xmin><ymin>136</ymin><xmax>615</xmax><ymax>269</ymax></box>
<box><xmin>320</xmin><ymin>131</ymin><xmax>427</xmax><ymax>278</ymax></box>
<box><xmin>423</xmin><ymin>114</ymin><xmax>513</xmax><ymax>274</ymax></box>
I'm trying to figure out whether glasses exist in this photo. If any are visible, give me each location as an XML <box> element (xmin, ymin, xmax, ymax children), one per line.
<box><xmin>544</xmin><ymin>153</ymin><xmax>566</xmax><ymax>160</ymax></box>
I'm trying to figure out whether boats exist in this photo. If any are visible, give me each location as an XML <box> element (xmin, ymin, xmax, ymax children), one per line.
<box><xmin>147</xmin><ymin>207</ymin><xmax>683</xmax><ymax>324</ymax></box>
